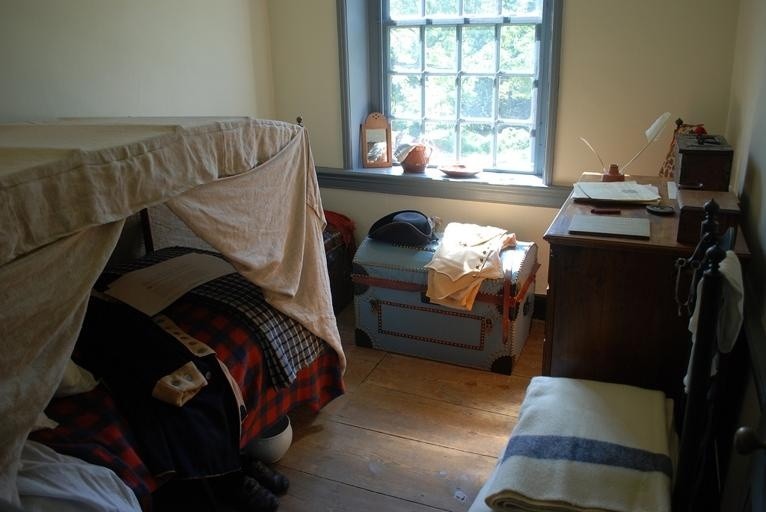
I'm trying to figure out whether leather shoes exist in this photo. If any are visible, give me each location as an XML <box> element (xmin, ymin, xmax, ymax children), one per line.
<box><xmin>242</xmin><ymin>458</ymin><xmax>289</xmax><ymax>492</ymax></box>
<box><xmin>238</xmin><ymin>474</ymin><xmax>281</xmax><ymax>511</ymax></box>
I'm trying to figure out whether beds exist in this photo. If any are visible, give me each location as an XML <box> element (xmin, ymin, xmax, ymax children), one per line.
<box><xmin>0</xmin><ymin>116</ymin><xmax>347</xmax><ymax>512</ymax></box>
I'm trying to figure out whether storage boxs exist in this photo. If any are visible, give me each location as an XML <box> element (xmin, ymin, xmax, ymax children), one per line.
<box><xmin>351</xmin><ymin>233</ymin><xmax>541</xmax><ymax>375</ymax></box>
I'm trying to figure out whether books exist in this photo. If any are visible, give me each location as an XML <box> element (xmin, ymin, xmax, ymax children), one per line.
<box><xmin>675</xmin><ymin>131</ymin><xmax>734</xmax><ymax>152</ymax></box>
<box><xmin>674</xmin><ymin>189</ymin><xmax>743</xmax><ymax>221</ymax></box>
<box><xmin>568</xmin><ymin>214</ymin><xmax>652</xmax><ymax>240</ymax></box>
<box><xmin>572</xmin><ymin>181</ymin><xmax>662</xmax><ymax>206</ymax></box>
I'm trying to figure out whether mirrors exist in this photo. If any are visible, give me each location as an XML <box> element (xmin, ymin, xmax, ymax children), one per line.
<box><xmin>360</xmin><ymin>112</ymin><xmax>392</xmax><ymax>168</ymax></box>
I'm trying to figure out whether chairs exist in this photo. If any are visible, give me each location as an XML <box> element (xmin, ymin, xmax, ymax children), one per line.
<box><xmin>467</xmin><ymin>197</ymin><xmax>725</xmax><ymax>512</ymax></box>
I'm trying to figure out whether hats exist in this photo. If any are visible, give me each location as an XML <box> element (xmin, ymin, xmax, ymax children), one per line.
<box><xmin>368</xmin><ymin>210</ymin><xmax>432</xmax><ymax>244</ymax></box>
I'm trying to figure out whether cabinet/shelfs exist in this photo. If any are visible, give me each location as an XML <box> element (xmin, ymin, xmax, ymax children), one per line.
<box><xmin>542</xmin><ymin>171</ymin><xmax>751</xmax><ymax>376</ymax></box>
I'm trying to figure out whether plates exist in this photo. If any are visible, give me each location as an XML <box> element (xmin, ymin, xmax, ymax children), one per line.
<box><xmin>439</xmin><ymin>166</ymin><xmax>482</xmax><ymax>178</ymax></box>
